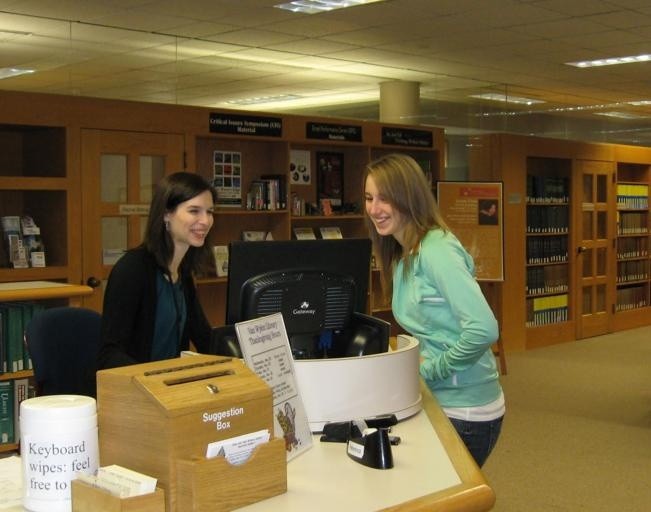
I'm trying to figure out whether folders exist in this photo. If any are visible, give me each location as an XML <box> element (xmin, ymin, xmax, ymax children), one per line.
<box><xmin>617</xmin><ymin>183</ymin><xmax>650</xmax><ymax>313</ymax></box>
<box><xmin>526</xmin><ymin>176</ymin><xmax>569</xmax><ymax>328</ymax></box>
<box><xmin>0</xmin><ymin>304</ymin><xmax>46</xmax><ymax>373</ymax></box>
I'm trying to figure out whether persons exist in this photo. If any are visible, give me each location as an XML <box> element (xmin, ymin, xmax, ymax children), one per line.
<box><xmin>364</xmin><ymin>153</ymin><xmax>506</xmax><ymax>468</ymax></box>
<box><xmin>102</xmin><ymin>171</ymin><xmax>235</xmax><ymax>366</ymax></box>
<box><xmin>479</xmin><ymin>200</ymin><xmax>498</xmax><ymax>225</ymax></box>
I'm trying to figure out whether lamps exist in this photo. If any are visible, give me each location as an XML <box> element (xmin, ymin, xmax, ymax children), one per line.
<box><xmin>379</xmin><ymin>80</ymin><xmax>422</xmax><ymax>126</ymax></box>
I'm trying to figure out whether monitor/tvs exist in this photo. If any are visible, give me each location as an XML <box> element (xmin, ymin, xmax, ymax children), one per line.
<box><xmin>226</xmin><ymin>238</ymin><xmax>372</xmax><ymax>359</ymax></box>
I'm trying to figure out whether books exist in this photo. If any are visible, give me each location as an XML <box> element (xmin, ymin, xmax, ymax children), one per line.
<box><xmin>77</xmin><ymin>463</ymin><xmax>159</xmax><ymax>499</ymax></box>
<box><xmin>0</xmin><ymin>301</ymin><xmax>47</xmax><ymax>445</ymax></box>
<box><xmin>616</xmin><ymin>185</ymin><xmax>649</xmax><ymax>310</ymax></box>
<box><xmin>250</xmin><ymin>173</ymin><xmax>286</xmax><ymax>211</ymax></box>
<box><xmin>526</xmin><ymin>174</ymin><xmax>570</xmax><ymax>328</ymax></box>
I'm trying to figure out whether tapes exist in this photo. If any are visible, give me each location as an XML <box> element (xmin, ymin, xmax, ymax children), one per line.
<box><xmin>361</xmin><ymin>428</ymin><xmax>378</xmax><ymax>438</ymax></box>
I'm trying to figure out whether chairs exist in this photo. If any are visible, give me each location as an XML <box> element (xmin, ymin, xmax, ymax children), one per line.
<box><xmin>25</xmin><ymin>309</ymin><xmax>110</xmax><ymax>399</ymax></box>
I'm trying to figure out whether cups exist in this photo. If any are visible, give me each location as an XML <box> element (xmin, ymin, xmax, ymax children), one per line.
<box><xmin>245</xmin><ymin>180</ymin><xmax>267</xmax><ymax>211</ymax></box>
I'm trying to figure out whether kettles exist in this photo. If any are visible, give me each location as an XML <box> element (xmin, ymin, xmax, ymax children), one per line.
<box><xmin>292</xmin><ymin>192</ymin><xmax>305</xmax><ymax>218</ymax></box>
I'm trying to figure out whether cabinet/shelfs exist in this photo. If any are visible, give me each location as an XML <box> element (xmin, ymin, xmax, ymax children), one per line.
<box><xmin>185</xmin><ymin>133</ymin><xmax>445</xmax><ymax>318</ymax></box>
<box><xmin>0</xmin><ymin>123</ymin><xmax>95</xmax><ymax>454</ymax></box>
<box><xmin>467</xmin><ymin>135</ymin><xmax>651</xmax><ymax>354</ymax></box>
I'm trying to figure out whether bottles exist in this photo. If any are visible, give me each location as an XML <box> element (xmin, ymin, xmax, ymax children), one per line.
<box><xmin>260</xmin><ymin>173</ymin><xmax>286</xmax><ymax>211</ymax></box>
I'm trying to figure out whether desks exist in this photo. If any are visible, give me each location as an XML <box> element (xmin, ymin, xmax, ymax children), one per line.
<box><xmin>3</xmin><ymin>333</ymin><xmax>494</xmax><ymax>512</ymax></box>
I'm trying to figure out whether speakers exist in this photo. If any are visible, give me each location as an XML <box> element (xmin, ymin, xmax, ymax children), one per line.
<box><xmin>335</xmin><ymin>312</ymin><xmax>391</xmax><ymax>357</ymax></box>
<box><xmin>207</xmin><ymin>325</ymin><xmax>243</xmax><ymax>358</ymax></box>
